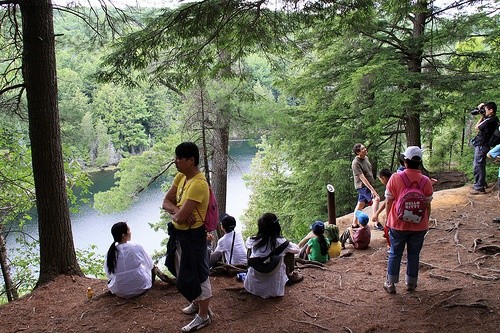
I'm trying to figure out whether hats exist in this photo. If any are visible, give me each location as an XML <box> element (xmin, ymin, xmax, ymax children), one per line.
<box><xmin>219</xmin><ymin>212</ymin><xmax>236</xmax><ymax>229</ymax></box>
<box><xmin>403</xmin><ymin>146</ymin><xmax>422</xmax><ymax>160</ymax></box>
<box><xmin>312</xmin><ymin>220</ymin><xmax>324</xmax><ymax>232</ymax></box>
<box><xmin>356</xmin><ymin>210</ymin><xmax>369</xmax><ymax>224</ymax></box>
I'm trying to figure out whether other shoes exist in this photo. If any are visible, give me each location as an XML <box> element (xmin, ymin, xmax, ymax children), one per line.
<box><xmin>406</xmin><ymin>284</ymin><xmax>415</xmax><ymax>290</ymax></box>
<box><xmin>286</xmin><ymin>272</ymin><xmax>304</xmax><ymax>285</ymax></box>
<box><xmin>346</xmin><ymin>238</ymin><xmax>351</xmax><ymax>243</ymax></box>
<box><xmin>473</xmin><ymin>183</ymin><xmax>488</xmax><ymax>189</ymax></box>
<box><xmin>383</xmin><ymin>282</ymin><xmax>395</xmax><ymax>293</ymax></box>
<box><xmin>470</xmin><ymin>190</ymin><xmax>485</xmax><ymax>194</ymax></box>
<box><xmin>341</xmin><ymin>243</ymin><xmax>346</xmax><ymax>249</ymax></box>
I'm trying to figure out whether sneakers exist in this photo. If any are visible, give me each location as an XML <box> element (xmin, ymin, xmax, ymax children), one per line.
<box><xmin>182</xmin><ymin>303</ymin><xmax>199</xmax><ymax>313</ymax></box>
<box><xmin>352</xmin><ymin>224</ymin><xmax>361</xmax><ymax>230</ymax></box>
<box><xmin>182</xmin><ymin>314</ymin><xmax>211</xmax><ymax>332</ymax></box>
<box><xmin>374</xmin><ymin>222</ymin><xmax>384</xmax><ymax>231</ymax></box>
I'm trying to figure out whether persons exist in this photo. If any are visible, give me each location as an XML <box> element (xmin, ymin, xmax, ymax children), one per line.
<box><xmin>383</xmin><ymin>146</ymin><xmax>433</xmax><ymax>294</ymax></box>
<box><xmin>162</xmin><ymin>142</ymin><xmax>212</xmax><ymax>333</ymax></box>
<box><xmin>304</xmin><ymin>221</ymin><xmax>330</xmax><ymax>264</ymax></box>
<box><xmin>205</xmin><ymin>230</ymin><xmax>224</xmax><ymax>276</ymax></box>
<box><xmin>396</xmin><ymin>154</ymin><xmax>438</xmax><ymax>188</ymax></box>
<box><xmin>339</xmin><ymin>210</ymin><xmax>371</xmax><ymax>250</ymax></box>
<box><xmin>486</xmin><ymin>121</ymin><xmax>500</xmax><ymax>230</ymax></box>
<box><xmin>372</xmin><ymin>168</ymin><xmax>395</xmax><ymax>254</ymax></box>
<box><xmin>322</xmin><ymin>224</ymin><xmax>341</xmax><ymax>260</ymax></box>
<box><xmin>210</xmin><ymin>213</ymin><xmax>249</xmax><ymax>278</ymax></box>
<box><xmin>243</xmin><ymin>212</ymin><xmax>304</xmax><ymax>298</ymax></box>
<box><xmin>104</xmin><ymin>222</ymin><xmax>155</xmax><ymax>299</ymax></box>
<box><xmin>469</xmin><ymin>101</ymin><xmax>500</xmax><ymax>194</ymax></box>
<box><xmin>352</xmin><ymin>143</ymin><xmax>389</xmax><ymax>231</ymax></box>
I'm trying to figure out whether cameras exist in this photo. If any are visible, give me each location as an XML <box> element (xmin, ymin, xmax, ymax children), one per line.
<box><xmin>471</xmin><ymin>108</ymin><xmax>485</xmax><ymax>115</ymax></box>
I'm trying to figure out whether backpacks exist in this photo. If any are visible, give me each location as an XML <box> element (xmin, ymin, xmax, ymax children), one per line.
<box><xmin>395</xmin><ymin>171</ymin><xmax>429</xmax><ymax>224</ymax></box>
<box><xmin>187</xmin><ymin>178</ymin><xmax>218</xmax><ymax>231</ymax></box>
